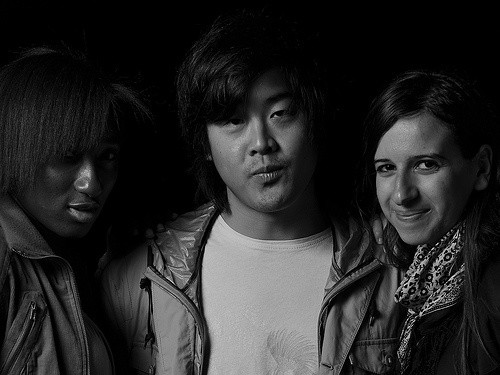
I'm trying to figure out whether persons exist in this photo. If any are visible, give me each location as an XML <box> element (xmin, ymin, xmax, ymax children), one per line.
<box><xmin>348</xmin><ymin>70</ymin><xmax>500</xmax><ymax>375</ymax></box>
<box><xmin>98</xmin><ymin>7</ymin><xmax>410</xmax><ymax>375</ymax></box>
<box><xmin>0</xmin><ymin>42</ymin><xmax>181</xmax><ymax>375</ymax></box>
